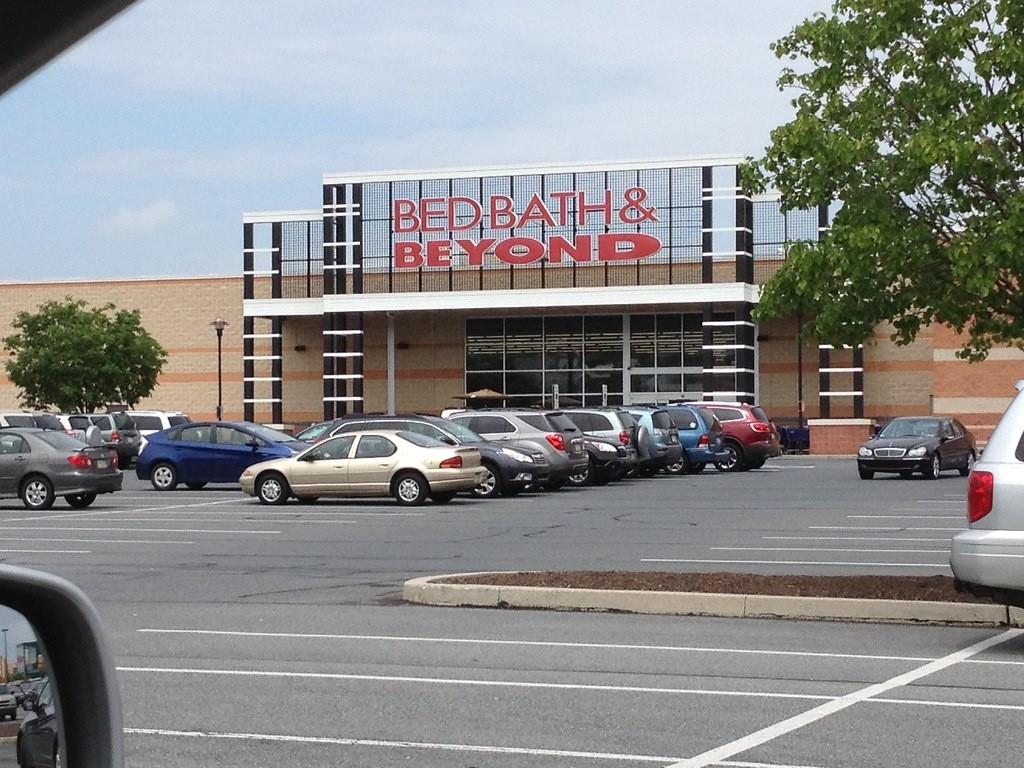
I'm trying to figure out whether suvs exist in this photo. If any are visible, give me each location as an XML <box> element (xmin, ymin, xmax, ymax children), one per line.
<box><xmin>311</xmin><ymin>411</ymin><xmax>549</xmax><ymax>498</ymax></box>
<box><xmin>53</xmin><ymin>413</ymin><xmax>107</xmax><ymax>446</ymax></box>
<box><xmin>674</xmin><ymin>400</ymin><xmax>783</xmax><ymax>474</ymax></box>
<box><xmin>617</xmin><ymin>403</ymin><xmax>683</xmax><ymax>478</ymax></box>
<box><xmin>123</xmin><ymin>408</ymin><xmax>202</xmax><ymax>459</ymax></box>
<box><xmin>654</xmin><ymin>401</ymin><xmax>731</xmax><ymax>476</ymax></box>
<box><xmin>442</xmin><ymin>406</ymin><xmax>590</xmax><ymax>494</ymax></box>
<box><xmin>556</xmin><ymin>405</ymin><xmax>650</xmax><ymax>487</ymax></box>
<box><xmin>70</xmin><ymin>409</ymin><xmax>142</xmax><ymax>472</ymax></box>
<box><xmin>0</xmin><ymin>412</ymin><xmax>73</xmax><ymax>449</ymax></box>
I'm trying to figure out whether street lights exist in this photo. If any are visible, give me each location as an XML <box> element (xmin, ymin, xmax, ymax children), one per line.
<box><xmin>209</xmin><ymin>316</ymin><xmax>231</xmax><ymax>422</ymax></box>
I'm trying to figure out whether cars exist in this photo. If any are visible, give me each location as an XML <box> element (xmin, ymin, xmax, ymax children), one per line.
<box><xmin>857</xmin><ymin>416</ymin><xmax>979</xmax><ymax>480</ymax></box>
<box><xmin>0</xmin><ymin>426</ymin><xmax>123</xmax><ymax>511</ymax></box>
<box><xmin>136</xmin><ymin>419</ymin><xmax>313</xmax><ymax>491</ymax></box>
<box><xmin>949</xmin><ymin>377</ymin><xmax>1024</xmax><ymax>591</ymax></box>
<box><xmin>0</xmin><ymin>674</ymin><xmax>64</xmax><ymax>768</ymax></box>
<box><xmin>238</xmin><ymin>429</ymin><xmax>484</xmax><ymax>506</ymax></box>
